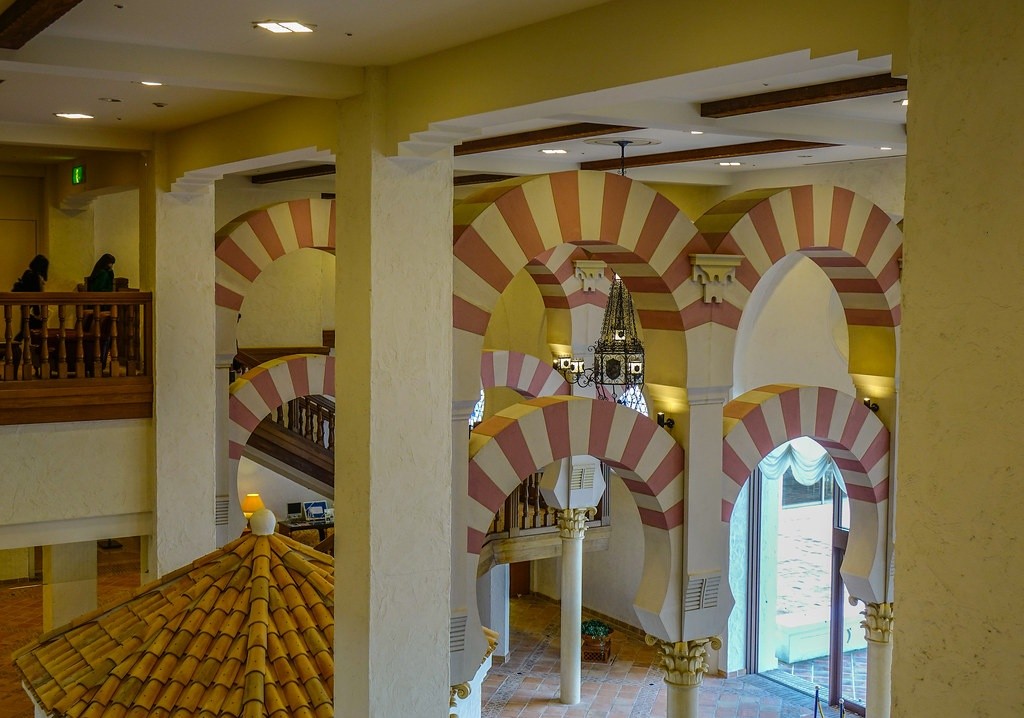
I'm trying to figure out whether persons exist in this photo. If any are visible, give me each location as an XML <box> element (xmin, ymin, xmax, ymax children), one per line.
<box><xmin>14</xmin><ymin>254</ymin><xmax>49</xmax><ymax>364</ymax></box>
<box><xmin>86</xmin><ymin>253</ymin><xmax>116</xmax><ymax>330</ymax></box>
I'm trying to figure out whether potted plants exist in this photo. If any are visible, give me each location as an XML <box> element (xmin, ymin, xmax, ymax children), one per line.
<box><xmin>582</xmin><ymin>620</ymin><xmax>614</xmax><ymax>663</ymax></box>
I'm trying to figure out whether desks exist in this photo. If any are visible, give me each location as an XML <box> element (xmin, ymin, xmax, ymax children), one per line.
<box><xmin>0</xmin><ymin>340</ymin><xmax>22</xmax><ymax>381</ymax></box>
<box><xmin>277</xmin><ymin>519</ymin><xmax>334</xmax><ymax>548</ymax></box>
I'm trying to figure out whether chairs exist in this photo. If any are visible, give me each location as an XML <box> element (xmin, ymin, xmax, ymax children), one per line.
<box><xmin>31</xmin><ymin>310</ymin><xmax>119</xmax><ymax>379</ymax></box>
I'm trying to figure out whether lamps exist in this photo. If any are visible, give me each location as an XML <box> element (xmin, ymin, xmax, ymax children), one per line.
<box><xmin>552</xmin><ymin>273</ymin><xmax>653</xmax><ymax>410</ymax></box>
<box><xmin>241</xmin><ymin>493</ymin><xmax>266</xmax><ymax>520</ymax></box>
<box><xmin>864</xmin><ymin>397</ymin><xmax>879</xmax><ymax>412</ymax></box>
<box><xmin>657</xmin><ymin>412</ymin><xmax>676</xmax><ymax>428</ymax></box>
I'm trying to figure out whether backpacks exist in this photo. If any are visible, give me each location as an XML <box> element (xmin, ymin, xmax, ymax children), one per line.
<box><xmin>11</xmin><ymin>271</ymin><xmax>41</xmax><ymax>293</ymax></box>
